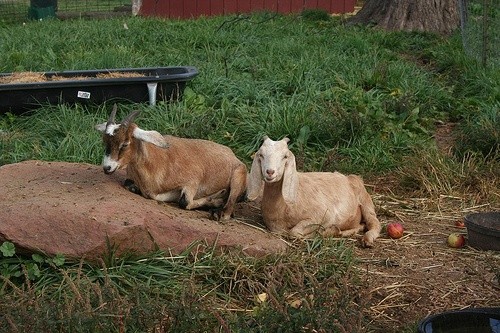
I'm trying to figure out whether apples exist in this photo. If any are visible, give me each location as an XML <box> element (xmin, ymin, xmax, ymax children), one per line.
<box><xmin>448</xmin><ymin>233</ymin><xmax>464</xmax><ymax>248</ymax></box>
<box><xmin>387</xmin><ymin>222</ymin><xmax>404</xmax><ymax>239</ymax></box>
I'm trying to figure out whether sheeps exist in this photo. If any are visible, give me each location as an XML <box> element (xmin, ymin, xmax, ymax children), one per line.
<box><xmin>94</xmin><ymin>103</ymin><xmax>246</xmax><ymax>224</ymax></box>
<box><xmin>246</xmin><ymin>137</ymin><xmax>381</xmax><ymax>248</ymax></box>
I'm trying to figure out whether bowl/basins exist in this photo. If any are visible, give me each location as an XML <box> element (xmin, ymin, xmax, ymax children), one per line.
<box><xmin>463</xmin><ymin>212</ymin><xmax>500</xmax><ymax>252</ymax></box>
